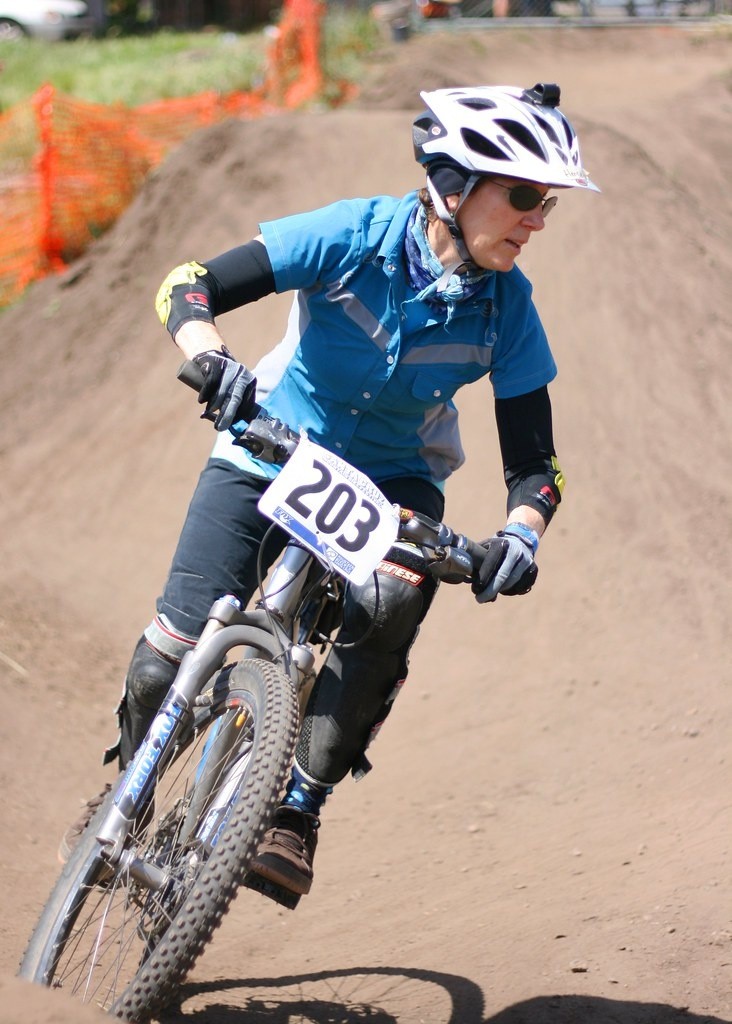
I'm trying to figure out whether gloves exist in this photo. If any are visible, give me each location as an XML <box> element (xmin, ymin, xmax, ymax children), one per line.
<box><xmin>472</xmin><ymin>522</ymin><xmax>540</xmax><ymax>604</ymax></box>
<box><xmin>193</xmin><ymin>345</ymin><xmax>257</xmax><ymax>432</ymax></box>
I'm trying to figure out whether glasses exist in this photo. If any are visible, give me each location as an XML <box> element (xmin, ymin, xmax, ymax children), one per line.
<box><xmin>488</xmin><ymin>180</ymin><xmax>559</xmax><ymax>218</ymax></box>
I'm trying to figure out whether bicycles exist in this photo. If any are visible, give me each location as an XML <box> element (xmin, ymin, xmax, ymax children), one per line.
<box><xmin>12</xmin><ymin>355</ymin><xmax>537</xmax><ymax>1021</ymax></box>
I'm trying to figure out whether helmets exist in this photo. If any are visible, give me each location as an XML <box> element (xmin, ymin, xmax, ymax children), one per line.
<box><xmin>411</xmin><ymin>82</ymin><xmax>602</xmax><ymax>194</ymax></box>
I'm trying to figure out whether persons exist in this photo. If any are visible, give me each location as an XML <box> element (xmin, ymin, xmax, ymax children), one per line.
<box><xmin>104</xmin><ymin>82</ymin><xmax>604</xmax><ymax>893</ymax></box>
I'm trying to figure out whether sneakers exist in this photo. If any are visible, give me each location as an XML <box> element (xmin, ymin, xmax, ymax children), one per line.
<box><xmin>250</xmin><ymin>809</ymin><xmax>321</xmax><ymax>894</ymax></box>
<box><xmin>59</xmin><ymin>782</ymin><xmax>156</xmax><ymax>881</ymax></box>
